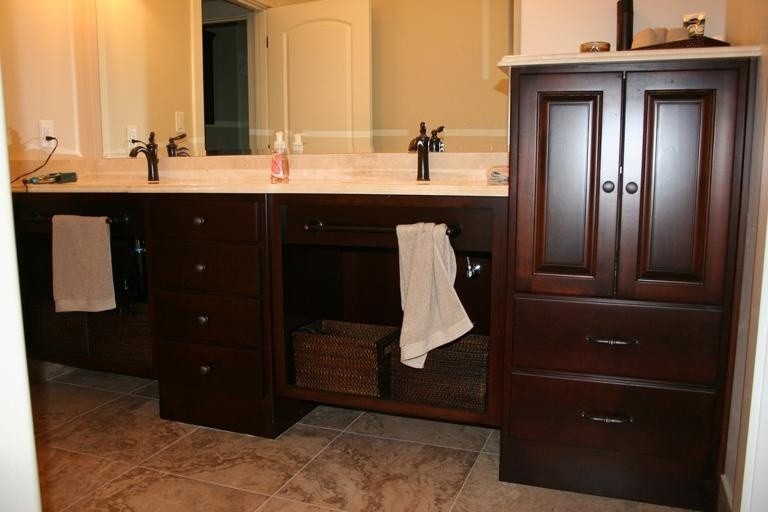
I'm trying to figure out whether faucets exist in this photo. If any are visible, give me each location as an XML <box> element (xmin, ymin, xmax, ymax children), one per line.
<box><xmin>130</xmin><ymin>132</ymin><xmax>161</xmax><ymax>182</ymax></box>
<box><xmin>166</xmin><ymin>133</ymin><xmax>190</xmax><ymax>157</ymax></box>
<box><xmin>409</xmin><ymin>121</ymin><xmax>432</xmax><ymax>180</ymax></box>
<box><xmin>429</xmin><ymin>126</ymin><xmax>447</xmax><ymax>152</ymax></box>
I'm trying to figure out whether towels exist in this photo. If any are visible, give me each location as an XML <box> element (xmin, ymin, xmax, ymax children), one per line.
<box><xmin>52</xmin><ymin>215</ymin><xmax>117</xmax><ymax>314</ymax></box>
<box><xmin>395</xmin><ymin>223</ymin><xmax>475</xmax><ymax>370</ymax></box>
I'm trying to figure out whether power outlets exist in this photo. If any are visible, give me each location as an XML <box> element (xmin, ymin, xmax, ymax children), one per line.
<box><xmin>128</xmin><ymin>127</ymin><xmax>138</xmax><ymax>152</ymax></box>
<box><xmin>40</xmin><ymin>120</ymin><xmax>54</xmax><ymax>149</ymax></box>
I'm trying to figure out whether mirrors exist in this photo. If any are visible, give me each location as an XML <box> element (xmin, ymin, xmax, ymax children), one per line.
<box><xmin>94</xmin><ymin>0</ymin><xmax>520</xmax><ymax>159</ymax></box>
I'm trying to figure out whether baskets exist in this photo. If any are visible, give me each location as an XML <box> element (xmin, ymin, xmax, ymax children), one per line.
<box><xmin>292</xmin><ymin>319</ymin><xmax>400</xmax><ymax>399</ymax></box>
<box><xmin>390</xmin><ymin>333</ymin><xmax>490</xmax><ymax>411</ymax></box>
<box><xmin>629</xmin><ymin>37</ymin><xmax>730</xmax><ymax>50</ymax></box>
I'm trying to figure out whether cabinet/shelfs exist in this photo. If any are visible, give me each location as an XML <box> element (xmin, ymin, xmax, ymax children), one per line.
<box><xmin>499</xmin><ymin>58</ymin><xmax>750</xmax><ymax>511</ymax></box>
<box><xmin>144</xmin><ymin>194</ymin><xmax>315</xmax><ymax>439</ymax></box>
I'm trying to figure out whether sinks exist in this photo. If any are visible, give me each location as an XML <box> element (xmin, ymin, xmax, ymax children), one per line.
<box><xmin>96</xmin><ymin>183</ymin><xmax>190</xmax><ymax>192</ymax></box>
<box><xmin>378</xmin><ymin>182</ymin><xmax>466</xmax><ymax>190</ymax></box>
